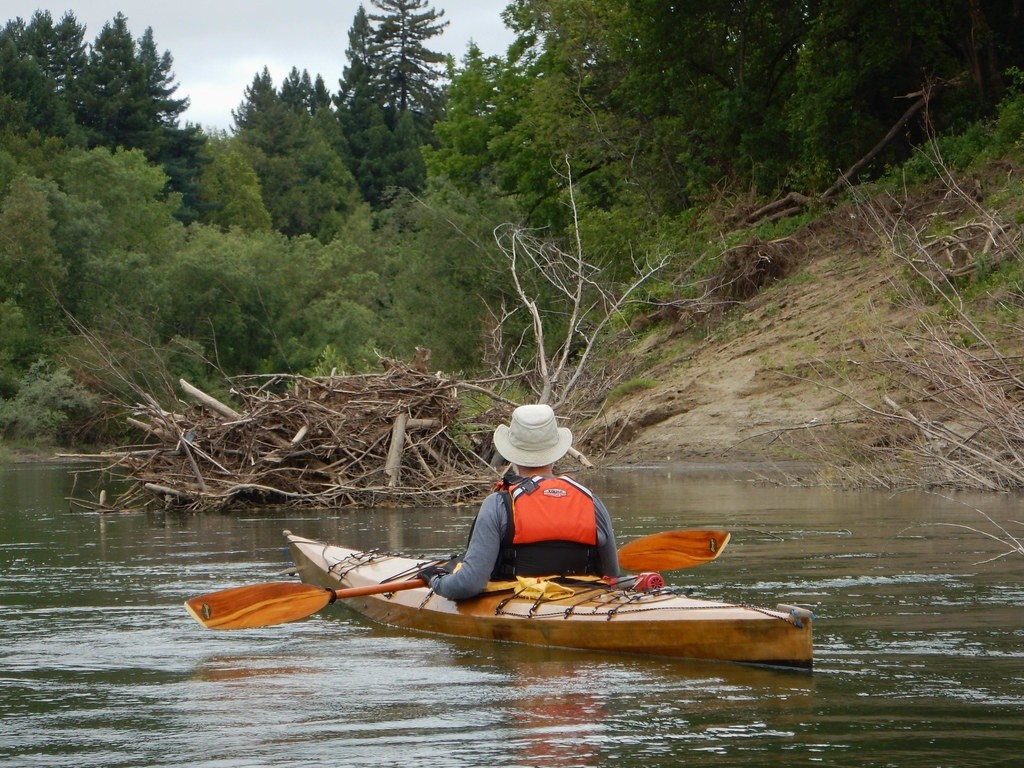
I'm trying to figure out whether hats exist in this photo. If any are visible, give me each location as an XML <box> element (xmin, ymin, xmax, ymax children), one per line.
<box><xmin>493</xmin><ymin>403</ymin><xmax>572</xmax><ymax>467</ymax></box>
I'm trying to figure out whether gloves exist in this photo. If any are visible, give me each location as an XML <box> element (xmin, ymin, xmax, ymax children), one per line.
<box><xmin>418</xmin><ymin>567</ymin><xmax>450</xmax><ymax>588</ymax></box>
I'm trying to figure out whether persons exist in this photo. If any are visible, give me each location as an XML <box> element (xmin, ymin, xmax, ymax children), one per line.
<box><xmin>417</xmin><ymin>404</ymin><xmax>619</xmax><ymax>599</ymax></box>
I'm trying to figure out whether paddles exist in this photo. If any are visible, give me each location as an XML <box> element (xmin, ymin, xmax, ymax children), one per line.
<box><xmin>182</xmin><ymin>528</ymin><xmax>732</xmax><ymax>631</ymax></box>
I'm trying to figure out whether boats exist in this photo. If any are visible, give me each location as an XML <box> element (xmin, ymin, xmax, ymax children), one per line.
<box><xmin>280</xmin><ymin>526</ymin><xmax>816</xmax><ymax>676</ymax></box>
